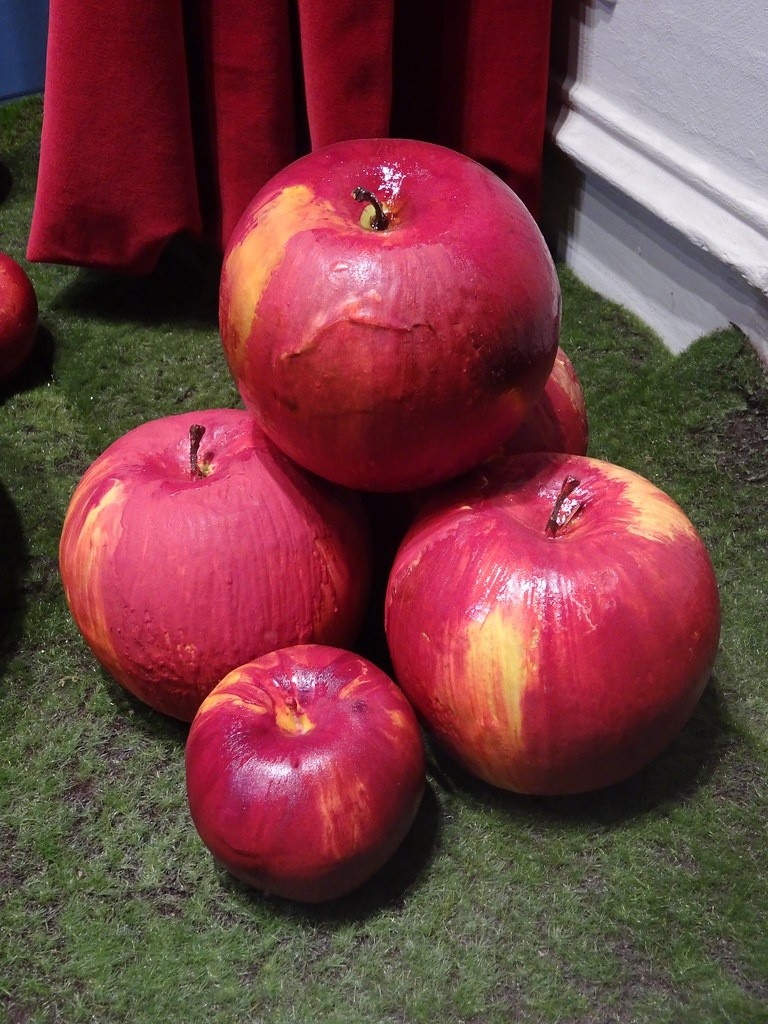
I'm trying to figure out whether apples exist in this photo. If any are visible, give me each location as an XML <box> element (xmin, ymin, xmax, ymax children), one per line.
<box><xmin>0</xmin><ymin>251</ymin><xmax>39</xmax><ymax>372</ymax></box>
<box><xmin>220</xmin><ymin>140</ymin><xmax>563</xmax><ymax>491</ymax></box>
<box><xmin>385</xmin><ymin>452</ymin><xmax>722</xmax><ymax>797</ymax></box>
<box><xmin>511</xmin><ymin>348</ymin><xmax>588</xmax><ymax>457</ymax></box>
<box><xmin>57</xmin><ymin>409</ymin><xmax>375</xmax><ymax>724</ymax></box>
<box><xmin>185</xmin><ymin>644</ymin><xmax>423</xmax><ymax>898</ymax></box>
